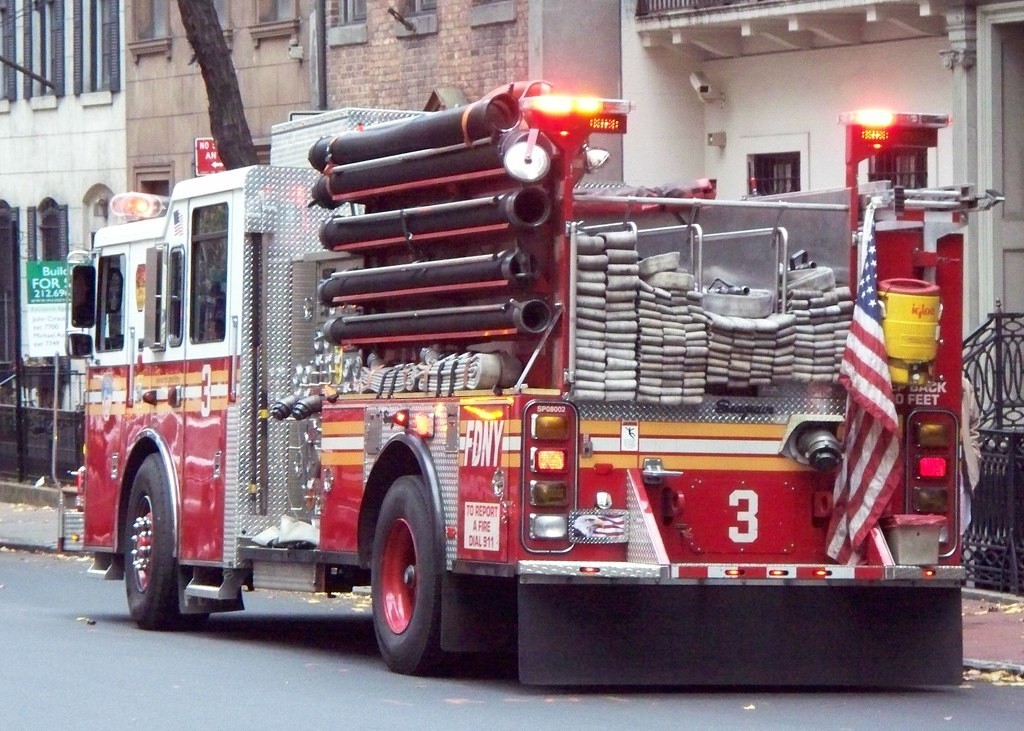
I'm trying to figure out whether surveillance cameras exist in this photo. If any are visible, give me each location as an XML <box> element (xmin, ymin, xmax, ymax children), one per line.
<box><xmin>690</xmin><ymin>70</ymin><xmax>711</xmax><ymax>94</ymax></box>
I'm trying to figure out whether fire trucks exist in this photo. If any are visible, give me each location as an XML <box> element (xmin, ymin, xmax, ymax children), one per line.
<box><xmin>57</xmin><ymin>80</ymin><xmax>1008</xmax><ymax>689</ymax></box>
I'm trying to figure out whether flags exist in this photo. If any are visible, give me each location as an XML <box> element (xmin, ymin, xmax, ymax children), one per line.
<box><xmin>824</xmin><ymin>214</ymin><xmax>903</xmax><ymax>565</ymax></box>
